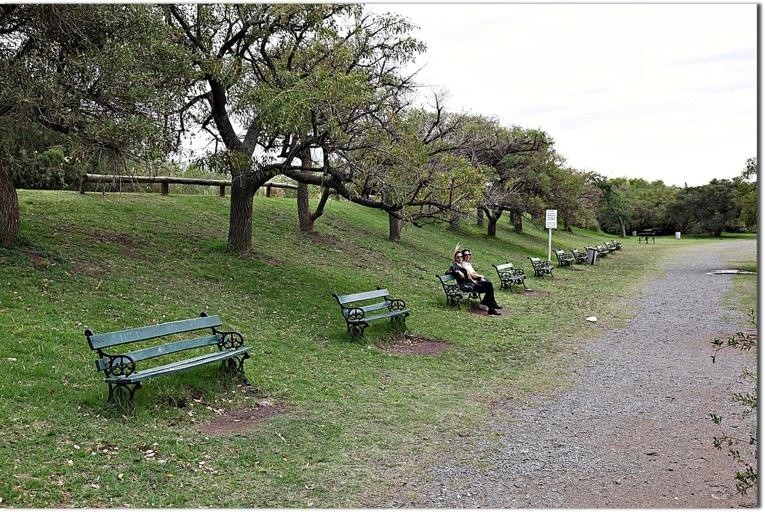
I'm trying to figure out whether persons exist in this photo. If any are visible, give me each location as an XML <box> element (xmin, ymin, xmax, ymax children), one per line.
<box><xmin>445</xmin><ymin>250</ymin><xmax>503</xmax><ymax>316</ymax></box>
<box><xmin>460</xmin><ymin>249</ymin><xmax>489</xmax><ymax>284</ymax></box>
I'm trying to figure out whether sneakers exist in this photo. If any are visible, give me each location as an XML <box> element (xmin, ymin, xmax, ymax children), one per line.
<box><xmin>479</xmin><ymin>304</ymin><xmax>489</xmax><ymax>310</ymax></box>
<box><xmin>491</xmin><ymin>306</ymin><xmax>502</xmax><ymax>309</ymax></box>
<box><xmin>488</xmin><ymin>310</ymin><xmax>501</xmax><ymax>315</ymax></box>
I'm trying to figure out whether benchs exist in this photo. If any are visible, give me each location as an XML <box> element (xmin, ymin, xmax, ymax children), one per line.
<box><xmin>492</xmin><ymin>262</ymin><xmax>528</xmax><ymax>292</ymax></box>
<box><xmin>332</xmin><ymin>286</ymin><xmax>412</xmax><ymax>338</ymax></box>
<box><xmin>84</xmin><ymin>313</ymin><xmax>254</xmax><ymax>413</ymax></box>
<box><xmin>528</xmin><ymin>256</ymin><xmax>555</xmax><ymax>279</ymax></box>
<box><xmin>436</xmin><ymin>272</ymin><xmax>483</xmax><ymax>309</ymax></box>
<box><xmin>553</xmin><ymin>239</ymin><xmax>622</xmax><ymax>267</ymax></box>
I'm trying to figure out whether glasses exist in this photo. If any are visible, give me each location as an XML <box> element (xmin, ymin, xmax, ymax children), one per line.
<box><xmin>456</xmin><ymin>256</ymin><xmax>463</xmax><ymax>258</ymax></box>
<box><xmin>465</xmin><ymin>254</ymin><xmax>471</xmax><ymax>256</ymax></box>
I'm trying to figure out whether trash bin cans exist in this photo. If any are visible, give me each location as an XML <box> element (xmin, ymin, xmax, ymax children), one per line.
<box><xmin>587</xmin><ymin>248</ymin><xmax>597</xmax><ymax>265</ymax></box>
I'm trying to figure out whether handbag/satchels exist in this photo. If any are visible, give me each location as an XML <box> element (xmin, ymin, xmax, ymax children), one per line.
<box><xmin>459</xmin><ymin>283</ymin><xmax>474</xmax><ymax>292</ymax></box>
